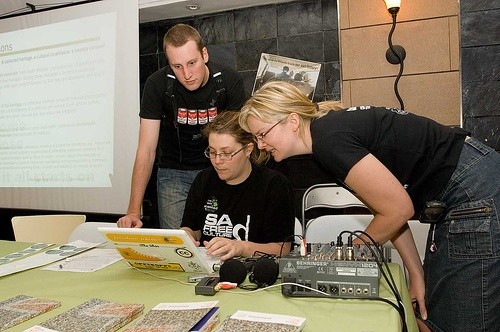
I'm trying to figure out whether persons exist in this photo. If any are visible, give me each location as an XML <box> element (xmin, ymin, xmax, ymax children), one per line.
<box><xmin>239</xmin><ymin>80</ymin><xmax>500</xmax><ymax>332</ymax></box>
<box><xmin>116</xmin><ymin>24</ymin><xmax>247</xmax><ymax>231</ymax></box>
<box><xmin>180</xmin><ymin>110</ymin><xmax>294</xmax><ymax>260</ymax></box>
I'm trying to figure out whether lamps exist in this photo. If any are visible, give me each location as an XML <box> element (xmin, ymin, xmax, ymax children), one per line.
<box><xmin>384</xmin><ymin>0</ymin><xmax>407</xmax><ymax>111</ymax></box>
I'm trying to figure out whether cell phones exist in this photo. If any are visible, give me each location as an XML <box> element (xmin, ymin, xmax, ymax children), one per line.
<box><xmin>188</xmin><ymin>275</ymin><xmax>204</xmax><ymax>283</ymax></box>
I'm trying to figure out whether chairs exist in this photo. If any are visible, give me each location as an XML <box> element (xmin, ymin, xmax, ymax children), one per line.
<box><xmin>11</xmin><ymin>215</ymin><xmax>87</xmax><ymax>243</ymax></box>
<box><xmin>301</xmin><ymin>182</ymin><xmax>389</xmax><ymax>263</ymax></box>
<box><xmin>67</xmin><ymin>221</ymin><xmax>118</xmax><ymax>243</ymax></box>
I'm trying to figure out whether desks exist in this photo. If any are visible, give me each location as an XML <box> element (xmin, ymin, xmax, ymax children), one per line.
<box><xmin>0</xmin><ymin>239</ymin><xmax>420</xmax><ymax>332</ymax></box>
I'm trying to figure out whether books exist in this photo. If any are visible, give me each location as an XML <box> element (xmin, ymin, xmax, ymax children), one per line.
<box><xmin>0</xmin><ymin>294</ymin><xmax>307</xmax><ymax>332</ymax></box>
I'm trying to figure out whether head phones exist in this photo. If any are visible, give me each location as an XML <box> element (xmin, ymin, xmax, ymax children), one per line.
<box><xmin>219</xmin><ymin>257</ymin><xmax>280</xmax><ymax>288</ymax></box>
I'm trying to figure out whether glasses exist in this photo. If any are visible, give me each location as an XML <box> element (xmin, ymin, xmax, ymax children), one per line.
<box><xmin>204</xmin><ymin>143</ymin><xmax>248</xmax><ymax>161</ymax></box>
<box><xmin>252</xmin><ymin>115</ymin><xmax>288</xmax><ymax>142</ymax></box>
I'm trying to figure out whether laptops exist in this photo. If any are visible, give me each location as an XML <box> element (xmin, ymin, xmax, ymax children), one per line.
<box><xmin>97</xmin><ymin>227</ymin><xmax>224</xmax><ymax>274</ymax></box>
